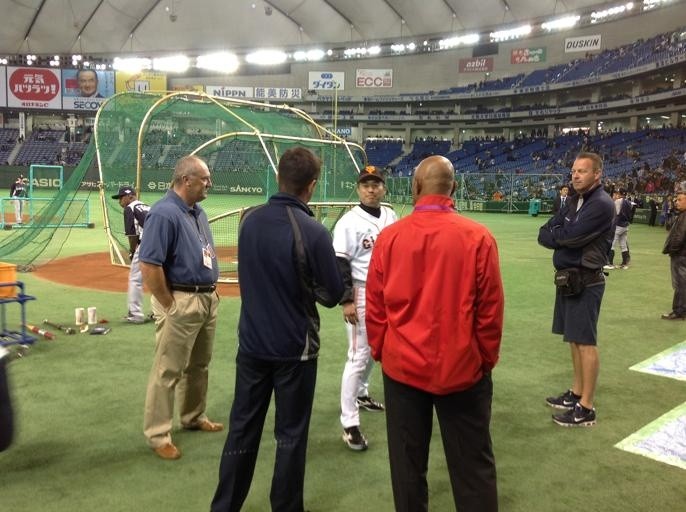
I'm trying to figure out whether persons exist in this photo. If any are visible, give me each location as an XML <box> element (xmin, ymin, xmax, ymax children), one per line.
<box><xmin>1</xmin><ymin>123</ymin><xmax>275</xmax><ymax>174</ymax></box>
<box><xmin>603</xmin><ymin>189</ymin><xmax>632</xmax><ymax>270</ymax></box>
<box><xmin>366</xmin><ymin>155</ymin><xmax>504</xmax><ymax>512</ymax></box>
<box><xmin>324</xmin><ymin>27</ymin><xmax>685</xmax><ymax>229</ymax></box>
<box><xmin>140</xmin><ymin>154</ymin><xmax>224</xmax><ymax>459</ymax></box>
<box><xmin>9</xmin><ymin>174</ymin><xmax>28</xmax><ymax>224</ymax></box>
<box><xmin>331</xmin><ymin>165</ymin><xmax>398</xmax><ymax>452</ymax></box>
<box><xmin>207</xmin><ymin>146</ymin><xmax>344</xmax><ymax>512</ymax></box>
<box><xmin>77</xmin><ymin>70</ymin><xmax>104</xmax><ymax>98</ymax></box>
<box><xmin>660</xmin><ymin>191</ymin><xmax>686</xmax><ymax>321</ymax></box>
<box><xmin>538</xmin><ymin>152</ymin><xmax>616</xmax><ymax>427</ymax></box>
<box><xmin>1</xmin><ymin>345</ymin><xmax>12</xmax><ymax>451</ymax></box>
<box><xmin>110</xmin><ymin>185</ymin><xmax>153</xmax><ymax>322</ymax></box>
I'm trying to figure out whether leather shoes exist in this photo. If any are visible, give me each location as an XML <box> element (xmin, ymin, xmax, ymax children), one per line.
<box><xmin>200</xmin><ymin>420</ymin><xmax>224</xmax><ymax>432</ymax></box>
<box><xmin>153</xmin><ymin>444</ymin><xmax>180</xmax><ymax>459</ymax></box>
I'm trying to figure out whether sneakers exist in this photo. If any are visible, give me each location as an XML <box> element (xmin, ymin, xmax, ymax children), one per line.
<box><xmin>342</xmin><ymin>426</ymin><xmax>368</xmax><ymax>451</ymax></box>
<box><xmin>546</xmin><ymin>389</ymin><xmax>577</xmax><ymax>410</ymax></box>
<box><xmin>552</xmin><ymin>402</ymin><xmax>596</xmax><ymax>427</ymax></box>
<box><xmin>357</xmin><ymin>396</ymin><xmax>385</xmax><ymax>412</ymax></box>
<box><xmin>602</xmin><ymin>264</ymin><xmax>629</xmax><ymax>270</ymax></box>
<box><xmin>662</xmin><ymin>312</ymin><xmax>686</xmax><ymax>320</ymax></box>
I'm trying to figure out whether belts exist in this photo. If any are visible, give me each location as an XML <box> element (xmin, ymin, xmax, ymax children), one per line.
<box><xmin>172</xmin><ymin>283</ymin><xmax>216</xmax><ymax>293</ymax></box>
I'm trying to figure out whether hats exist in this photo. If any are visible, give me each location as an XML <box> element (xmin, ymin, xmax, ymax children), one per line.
<box><xmin>112</xmin><ymin>187</ymin><xmax>136</xmax><ymax>199</ymax></box>
<box><xmin>357</xmin><ymin>166</ymin><xmax>385</xmax><ymax>185</ymax></box>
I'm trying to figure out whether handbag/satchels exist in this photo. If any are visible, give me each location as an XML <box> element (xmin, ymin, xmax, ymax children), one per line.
<box><xmin>554</xmin><ymin>267</ymin><xmax>583</xmax><ymax>296</ymax></box>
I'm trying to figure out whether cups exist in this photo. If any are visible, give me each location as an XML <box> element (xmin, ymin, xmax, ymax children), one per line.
<box><xmin>75</xmin><ymin>307</ymin><xmax>97</xmax><ymax>325</ymax></box>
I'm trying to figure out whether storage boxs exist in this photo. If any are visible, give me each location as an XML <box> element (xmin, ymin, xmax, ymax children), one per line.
<box><xmin>0</xmin><ymin>262</ymin><xmax>17</xmax><ymax>299</ymax></box>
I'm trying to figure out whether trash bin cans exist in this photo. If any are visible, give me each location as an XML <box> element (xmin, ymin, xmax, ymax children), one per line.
<box><xmin>528</xmin><ymin>198</ymin><xmax>541</xmax><ymax>217</ymax></box>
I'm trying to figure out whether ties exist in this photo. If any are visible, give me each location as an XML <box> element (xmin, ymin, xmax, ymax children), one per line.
<box><xmin>562</xmin><ymin>198</ymin><xmax>564</xmax><ymax>208</ymax></box>
